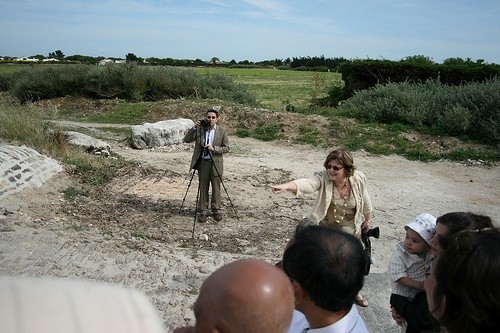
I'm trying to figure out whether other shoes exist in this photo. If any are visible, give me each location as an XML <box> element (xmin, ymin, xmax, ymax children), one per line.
<box><xmin>214</xmin><ymin>212</ymin><xmax>222</xmax><ymax>221</ymax></box>
<box><xmin>197</xmin><ymin>212</ymin><xmax>207</xmax><ymax>222</ymax></box>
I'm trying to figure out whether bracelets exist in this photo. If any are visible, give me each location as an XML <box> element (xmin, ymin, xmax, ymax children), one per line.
<box><xmin>365</xmin><ymin>219</ymin><xmax>371</xmax><ymax>222</ymax></box>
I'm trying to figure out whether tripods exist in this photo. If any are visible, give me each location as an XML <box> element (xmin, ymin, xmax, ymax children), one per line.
<box><xmin>179</xmin><ymin>127</ymin><xmax>240</xmax><ymax>239</ymax></box>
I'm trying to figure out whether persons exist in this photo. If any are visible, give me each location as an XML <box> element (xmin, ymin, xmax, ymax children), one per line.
<box><xmin>274</xmin><ymin>222</ymin><xmax>368</xmax><ymax>333</ymax></box>
<box><xmin>389</xmin><ymin>212</ymin><xmax>500</xmax><ymax>333</ymax></box>
<box><xmin>270</xmin><ymin>149</ymin><xmax>372</xmax><ymax>307</ymax></box>
<box><xmin>172</xmin><ymin>258</ymin><xmax>295</xmax><ymax>333</ymax></box>
<box><xmin>183</xmin><ymin>109</ymin><xmax>229</xmax><ymax>223</ymax></box>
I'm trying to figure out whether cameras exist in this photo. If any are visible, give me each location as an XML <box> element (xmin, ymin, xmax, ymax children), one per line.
<box><xmin>198</xmin><ymin>119</ymin><xmax>211</xmax><ymax>127</ymax></box>
<box><xmin>361</xmin><ymin>226</ymin><xmax>380</xmax><ymax>241</ymax></box>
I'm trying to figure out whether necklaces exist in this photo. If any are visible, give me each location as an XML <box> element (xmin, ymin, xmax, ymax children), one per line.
<box><xmin>333</xmin><ymin>176</ymin><xmax>349</xmax><ymax>224</ymax></box>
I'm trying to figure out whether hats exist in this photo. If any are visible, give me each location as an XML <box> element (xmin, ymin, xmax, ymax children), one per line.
<box><xmin>404</xmin><ymin>212</ymin><xmax>437</xmax><ymax>245</ymax></box>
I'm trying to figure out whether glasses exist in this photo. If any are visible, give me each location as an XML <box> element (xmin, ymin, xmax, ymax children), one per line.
<box><xmin>455</xmin><ymin>226</ymin><xmax>492</xmax><ymax>252</ymax></box>
<box><xmin>207</xmin><ymin>115</ymin><xmax>216</xmax><ymax>119</ymax></box>
<box><xmin>326</xmin><ymin>164</ymin><xmax>344</xmax><ymax>171</ymax></box>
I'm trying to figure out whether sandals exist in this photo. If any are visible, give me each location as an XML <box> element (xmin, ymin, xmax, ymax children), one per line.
<box><xmin>355</xmin><ymin>294</ymin><xmax>369</xmax><ymax>307</ymax></box>
